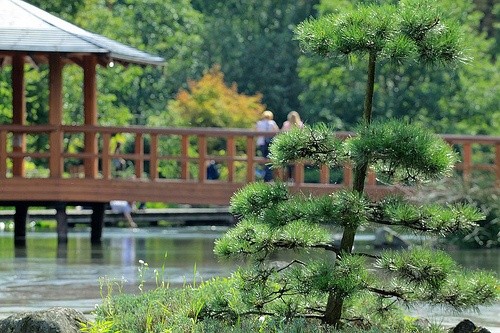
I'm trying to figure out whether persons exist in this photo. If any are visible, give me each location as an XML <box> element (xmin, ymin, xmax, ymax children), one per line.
<box><xmin>277</xmin><ymin>110</ymin><xmax>305</xmax><ymax>132</ymax></box>
<box><xmin>255</xmin><ymin>110</ymin><xmax>279</xmax><ymax>182</ymax></box>
